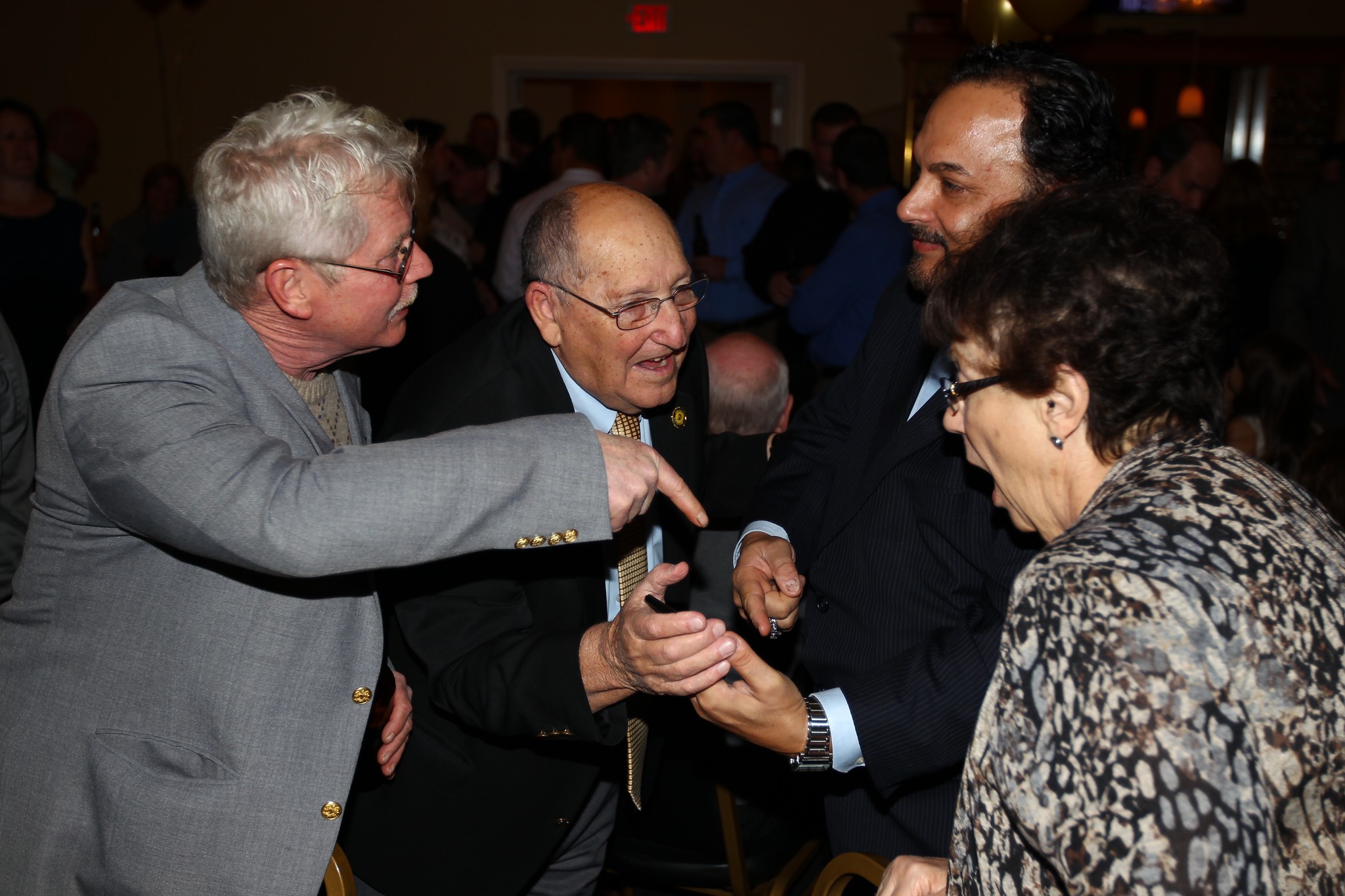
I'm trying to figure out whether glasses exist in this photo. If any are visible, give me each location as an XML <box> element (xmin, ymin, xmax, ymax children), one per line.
<box><xmin>937</xmin><ymin>370</ymin><xmax>1004</xmax><ymax>416</ymax></box>
<box><xmin>252</xmin><ymin>198</ymin><xmax>418</xmax><ymax>286</ymax></box>
<box><xmin>537</xmin><ymin>263</ymin><xmax>712</xmax><ymax>331</ymax></box>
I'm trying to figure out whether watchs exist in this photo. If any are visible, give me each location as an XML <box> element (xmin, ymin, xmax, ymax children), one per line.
<box><xmin>784</xmin><ymin>695</ymin><xmax>833</xmax><ymax>775</ymax></box>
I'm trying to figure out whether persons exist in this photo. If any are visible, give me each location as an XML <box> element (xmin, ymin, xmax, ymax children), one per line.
<box><xmin>2</xmin><ymin>87</ymin><xmax>713</xmax><ymax>896</ymax></box>
<box><xmin>336</xmin><ymin>181</ymin><xmax>738</xmax><ymax>895</ymax></box>
<box><xmin>685</xmin><ymin>35</ymin><xmax>1156</xmax><ymax>895</ymax></box>
<box><xmin>3</xmin><ymin>71</ymin><xmax>1343</xmax><ymax>465</ymax></box>
<box><xmin>869</xmin><ymin>180</ymin><xmax>1344</xmax><ymax>894</ymax></box>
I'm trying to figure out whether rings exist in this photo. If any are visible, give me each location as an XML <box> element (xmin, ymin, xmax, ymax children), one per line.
<box><xmin>765</xmin><ymin>617</ymin><xmax>782</xmax><ymax>640</ymax></box>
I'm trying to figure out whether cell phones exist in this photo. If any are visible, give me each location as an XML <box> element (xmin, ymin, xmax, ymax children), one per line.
<box><xmin>643</xmin><ymin>593</ymin><xmax>745</xmax><ymax>687</ymax></box>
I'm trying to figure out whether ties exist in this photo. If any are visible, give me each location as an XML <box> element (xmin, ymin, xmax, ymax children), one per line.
<box><xmin>610</xmin><ymin>412</ymin><xmax>648</xmax><ymax>811</ymax></box>
